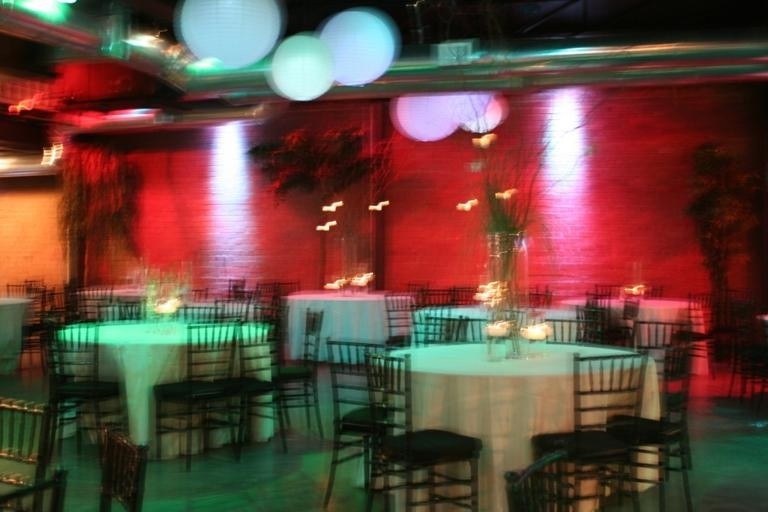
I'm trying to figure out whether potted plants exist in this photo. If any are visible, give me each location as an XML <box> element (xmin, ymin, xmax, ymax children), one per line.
<box><xmin>442</xmin><ymin>39</ymin><xmax>608</xmax><ymax>364</ymax></box>
